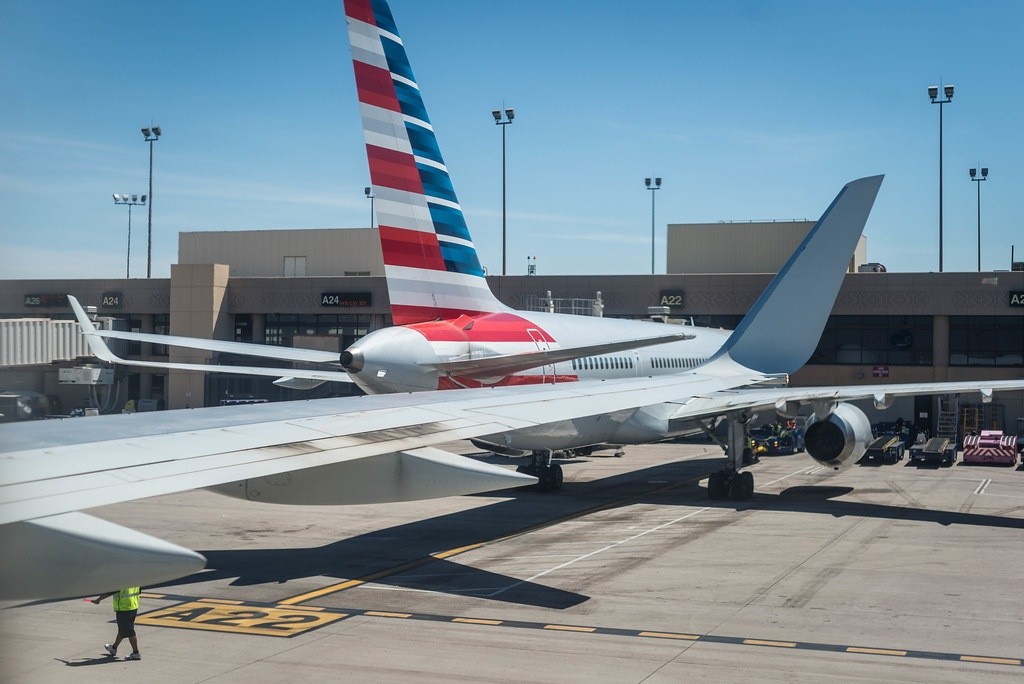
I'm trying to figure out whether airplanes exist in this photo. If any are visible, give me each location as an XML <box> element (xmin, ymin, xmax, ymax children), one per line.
<box><xmin>67</xmin><ymin>0</ymin><xmax>1024</xmax><ymax>501</ymax></box>
<box><xmin>0</xmin><ymin>174</ymin><xmax>886</xmax><ymax>601</ymax></box>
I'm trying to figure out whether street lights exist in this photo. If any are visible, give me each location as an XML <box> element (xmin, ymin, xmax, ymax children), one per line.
<box><xmin>928</xmin><ymin>75</ymin><xmax>955</xmax><ymax>272</ymax></box>
<box><xmin>645</xmin><ymin>178</ymin><xmax>662</xmax><ymax>274</ymax></box>
<box><xmin>113</xmin><ymin>193</ymin><xmax>147</xmax><ymax>279</ymax></box>
<box><xmin>142</xmin><ymin>126</ymin><xmax>161</xmax><ymax>279</ymax></box>
<box><xmin>364</xmin><ymin>187</ymin><xmax>374</xmax><ymax>228</ymax></box>
<box><xmin>493</xmin><ymin>108</ymin><xmax>514</xmax><ymax>275</ymax></box>
<box><xmin>969</xmin><ymin>160</ymin><xmax>988</xmax><ymax>272</ymax></box>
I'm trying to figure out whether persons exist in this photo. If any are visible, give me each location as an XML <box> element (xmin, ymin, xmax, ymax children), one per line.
<box><xmin>90</xmin><ymin>586</ymin><xmax>142</xmax><ymax>660</ymax></box>
<box><xmin>786</xmin><ymin>418</ymin><xmax>796</xmax><ymax>430</ymax></box>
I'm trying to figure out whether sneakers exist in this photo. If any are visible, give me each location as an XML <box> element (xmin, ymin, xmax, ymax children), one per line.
<box><xmin>105</xmin><ymin>644</ymin><xmax>117</xmax><ymax>656</ymax></box>
<box><xmin>125</xmin><ymin>652</ymin><xmax>141</xmax><ymax>660</ymax></box>
